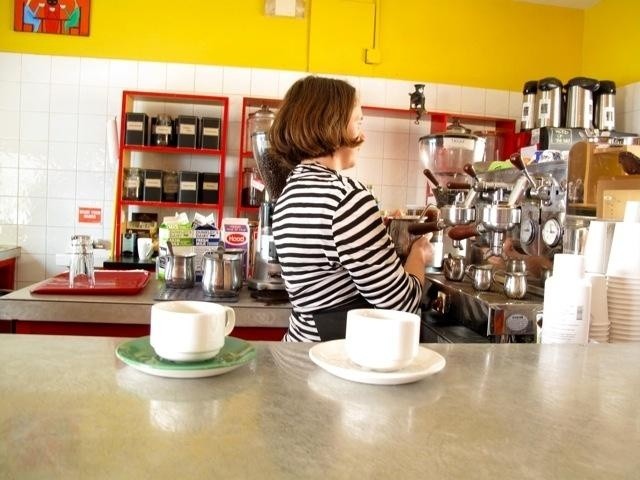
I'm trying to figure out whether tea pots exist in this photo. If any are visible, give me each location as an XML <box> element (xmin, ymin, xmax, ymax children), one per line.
<box><xmin>154</xmin><ymin>252</ymin><xmax>197</xmax><ymax>289</ymax></box>
<box><xmin>520</xmin><ymin>76</ymin><xmax>615</xmax><ymax>132</ymax></box>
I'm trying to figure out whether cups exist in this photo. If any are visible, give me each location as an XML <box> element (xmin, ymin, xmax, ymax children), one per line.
<box><xmin>537</xmin><ymin>201</ymin><xmax>640</xmax><ymax>346</ymax></box>
<box><xmin>441</xmin><ymin>255</ymin><xmax>529</xmax><ymax>300</ymax></box>
<box><xmin>135</xmin><ymin>236</ymin><xmax>153</xmax><ymax>260</ymax></box>
<box><xmin>337</xmin><ymin>402</ymin><xmax>413</xmax><ymax>448</ymax></box>
<box><xmin>68</xmin><ymin>234</ymin><xmax>96</xmax><ymax>289</ymax></box>
<box><xmin>199</xmin><ymin>249</ymin><xmax>243</xmax><ymax>298</ymax></box>
<box><xmin>146</xmin><ymin>396</ymin><xmax>222</xmax><ymax>438</ymax></box>
<box><xmin>344</xmin><ymin>309</ymin><xmax>421</xmax><ymax>374</ymax></box>
<box><xmin>150</xmin><ymin>298</ymin><xmax>236</xmax><ymax>363</ymax></box>
<box><xmin>388</xmin><ymin>213</ymin><xmax>429</xmax><ymax>258</ymax></box>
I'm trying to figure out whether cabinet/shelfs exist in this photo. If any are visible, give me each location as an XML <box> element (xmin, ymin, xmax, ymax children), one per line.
<box><xmin>113</xmin><ymin>91</ymin><xmax>229</xmax><ymax>268</ymax></box>
<box><xmin>236</xmin><ymin>97</ymin><xmax>516</xmax><ymax>231</ymax></box>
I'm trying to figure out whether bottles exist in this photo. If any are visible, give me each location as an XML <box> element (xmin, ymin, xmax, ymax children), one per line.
<box><xmin>123</xmin><ymin>166</ymin><xmax>140</xmax><ymax>199</ymax></box>
<box><xmin>245</xmin><ymin>102</ymin><xmax>276</xmax><ymax>154</ymax></box>
<box><xmin>151</xmin><ymin>112</ymin><xmax>173</xmax><ymax>144</ymax></box>
<box><xmin>242</xmin><ymin>166</ymin><xmax>265</xmax><ymax>209</ymax></box>
<box><xmin>161</xmin><ymin>169</ymin><xmax>178</xmax><ymax>201</ymax></box>
<box><xmin>409</xmin><ymin>83</ymin><xmax>425</xmax><ymax>109</ymax></box>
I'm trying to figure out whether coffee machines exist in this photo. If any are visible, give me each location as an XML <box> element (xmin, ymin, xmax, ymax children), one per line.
<box><xmin>419</xmin><ymin>119</ymin><xmax>488</xmax><ymax>271</ymax></box>
<box><xmin>246</xmin><ymin>129</ymin><xmax>297</xmax><ymax>293</ymax></box>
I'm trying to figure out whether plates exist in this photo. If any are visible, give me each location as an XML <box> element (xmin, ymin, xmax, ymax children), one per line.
<box><xmin>306</xmin><ymin>366</ymin><xmax>450</xmax><ymax>408</ymax></box>
<box><xmin>116</xmin><ymin>365</ymin><xmax>260</xmax><ymax>403</ymax></box>
<box><xmin>308</xmin><ymin>338</ymin><xmax>447</xmax><ymax>387</ymax></box>
<box><xmin>113</xmin><ymin>334</ymin><xmax>259</xmax><ymax>377</ymax></box>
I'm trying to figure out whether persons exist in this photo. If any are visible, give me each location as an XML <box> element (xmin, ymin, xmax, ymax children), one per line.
<box><xmin>267</xmin><ymin>75</ymin><xmax>435</xmax><ymax>342</ymax></box>
